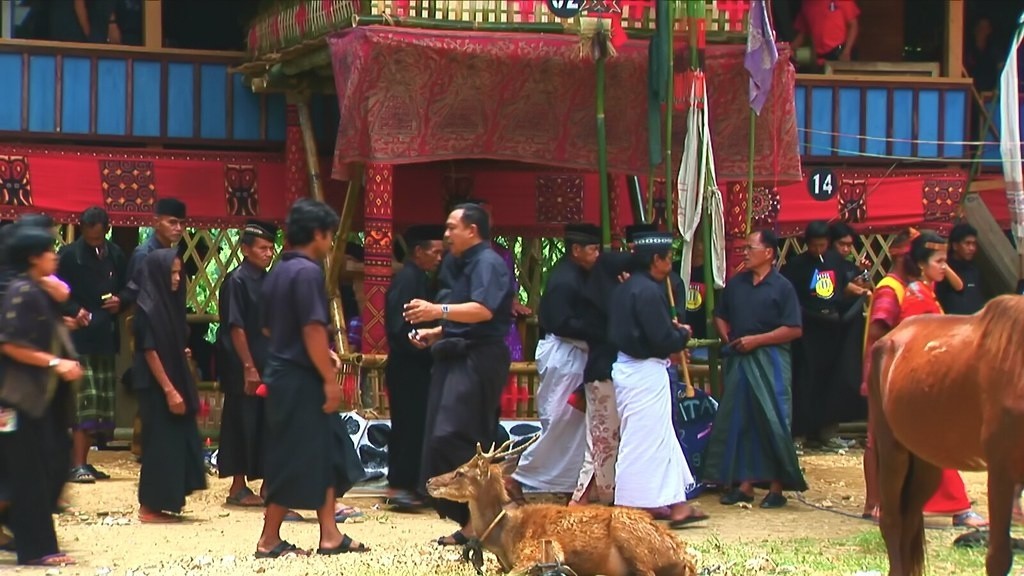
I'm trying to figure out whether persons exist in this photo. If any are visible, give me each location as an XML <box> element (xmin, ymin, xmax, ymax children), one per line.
<box><xmin>253</xmin><ymin>196</ymin><xmax>372</xmax><ymax>559</ymax></box>
<box><xmin>933</xmin><ymin>224</ymin><xmax>990</xmax><ymax>315</ymax></box>
<box><xmin>605</xmin><ymin>231</ymin><xmax>709</xmax><ymax>527</ymax></box>
<box><xmin>509</xmin><ymin>221</ymin><xmax>601</xmax><ymax>508</ymax></box>
<box><xmin>133</xmin><ymin>247</ymin><xmax>208</xmax><ymax>522</ymax></box>
<box><xmin>626</xmin><ymin>224</ymin><xmax>687</xmax><ymax>326</ymax></box>
<box><xmin>382</xmin><ymin>223</ymin><xmax>446</xmax><ymax>510</ymax></box>
<box><xmin>859</xmin><ymin>226</ymin><xmax>922</xmax><ymax>520</ymax></box>
<box><xmin>56</xmin><ymin>206</ymin><xmax>128</xmax><ymax>483</ymax></box>
<box><xmin>828</xmin><ymin>220</ymin><xmax>873</xmax><ymax>318</ymax></box>
<box><xmin>402</xmin><ymin>202</ymin><xmax>516</xmax><ymax>546</ymax></box>
<box><xmin>566</xmin><ymin>251</ymin><xmax>638</xmax><ymax>507</ymax></box>
<box><xmin>778</xmin><ymin>219</ymin><xmax>873</xmax><ymax>455</ymax></box>
<box><xmin>0</xmin><ymin>227</ymin><xmax>86</xmax><ymax>566</ymax></box>
<box><xmin>1</xmin><ymin>215</ymin><xmax>76</xmax><ymax>514</ymax></box>
<box><xmin>127</xmin><ymin>197</ymin><xmax>186</xmax><ymax>464</ymax></box>
<box><xmin>216</xmin><ymin>218</ymin><xmax>277</xmax><ymax>505</ymax></box>
<box><xmin>709</xmin><ymin>228</ymin><xmax>809</xmax><ymax>508</ymax></box>
<box><xmin>896</xmin><ymin>230</ymin><xmax>989</xmax><ymax>529</ymax></box>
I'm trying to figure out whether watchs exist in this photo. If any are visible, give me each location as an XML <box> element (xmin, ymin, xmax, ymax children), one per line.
<box><xmin>47</xmin><ymin>356</ymin><xmax>60</xmax><ymax>369</ymax></box>
<box><xmin>441</xmin><ymin>304</ymin><xmax>449</xmax><ymax>319</ymax></box>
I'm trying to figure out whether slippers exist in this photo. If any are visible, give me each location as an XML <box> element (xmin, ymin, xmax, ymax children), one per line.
<box><xmin>720</xmin><ymin>488</ymin><xmax>754</xmax><ymax>504</ymax></box>
<box><xmin>141</xmin><ymin>487</ymin><xmax>363</xmax><ymax>523</ymax></box>
<box><xmin>317</xmin><ymin>533</ymin><xmax>371</xmax><ymax>554</ymax></box>
<box><xmin>437</xmin><ymin>530</ymin><xmax>469</xmax><ymax>544</ymax></box>
<box><xmin>67</xmin><ymin>464</ymin><xmax>110</xmax><ymax>482</ymax></box>
<box><xmin>670</xmin><ymin>507</ymin><xmax>709</xmax><ymax>527</ymax></box>
<box><xmin>763</xmin><ymin>493</ymin><xmax>787</xmax><ymax>509</ymax></box>
<box><xmin>254</xmin><ymin>540</ymin><xmax>308</xmax><ymax>559</ymax></box>
<box><xmin>0</xmin><ymin>503</ymin><xmax>84</xmax><ymax>565</ymax></box>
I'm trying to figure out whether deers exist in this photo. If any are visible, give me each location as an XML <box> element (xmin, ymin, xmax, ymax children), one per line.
<box><xmin>425</xmin><ymin>429</ymin><xmax>697</xmax><ymax>575</ymax></box>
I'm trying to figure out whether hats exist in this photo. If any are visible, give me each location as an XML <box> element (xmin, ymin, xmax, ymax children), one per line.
<box><xmin>154</xmin><ymin>198</ymin><xmax>186</xmax><ymax>219</ymax></box>
<box><xmin>626</xmin><ymin>225</ymin><xmax>656</xmax><ymax>242</ymax></box>
<box><xmin>632</xmin><ymin>231</ymin><xmax>673</xmax><ymax>252</ymax></box>
<box><xmin>565</xmin><ymin>224</ymin><xmax>602</xmax><ymax>245</ymax></box>
<box><xmin>245</xmin><ymin>219</ymin><xmax>277</xmax><ymax>243</ymax></box>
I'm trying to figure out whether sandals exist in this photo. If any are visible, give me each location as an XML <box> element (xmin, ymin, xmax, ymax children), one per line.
<box><xmin>953</xmin><ymin>511</ymin><xmax>990</xmax><ymax>529</ymax></box>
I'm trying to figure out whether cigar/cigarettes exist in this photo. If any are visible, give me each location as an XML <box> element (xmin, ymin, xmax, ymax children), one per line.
<box><xmin>861</xmin><ymin>252</ymin><xmax>867</xmax><ymax>259</ymax></box>
<box><xmin>95</xmin><ymin>247</ymin><xmax>99</xmax><ymax>255</ymax></box>
<box><xmin>819</xmin><ymin>254</ymin><xmax>825</xmax><ymax>263</ymax></box>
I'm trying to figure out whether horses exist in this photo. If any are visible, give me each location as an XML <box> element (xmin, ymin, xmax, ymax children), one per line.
<box><xmin>867</xmin><ymin>293</ymin><xmax>1024</xmax><ymax>576</ymax></box>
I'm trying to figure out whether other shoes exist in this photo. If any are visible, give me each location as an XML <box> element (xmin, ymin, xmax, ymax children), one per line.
<box><xmin>390</xmin><ymin>494</ymin><xmax>423</xmax><ymax>510</ymax></box>
